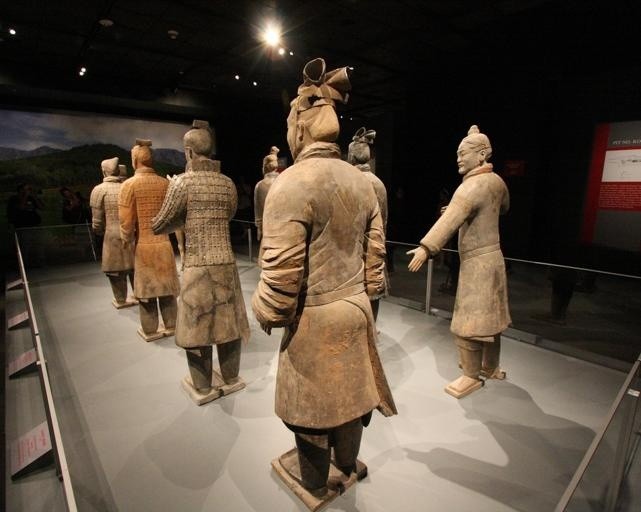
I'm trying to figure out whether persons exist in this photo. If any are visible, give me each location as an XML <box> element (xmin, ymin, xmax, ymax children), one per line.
<box><xmin>7</xmin><ymin>176</ymin><xmax>45</xmax><ymax>265</ymax></box>
<box><xmin>117</xmin><ymin>139</ymin><xmax>181</xmax><ymax>343</ymax></box>
<box><xmin>250</xmin><ymin>58</ymin><xmax>400</xmax><ymax>512</ymax></box>
<box><xmin>253</xmin><ymin>146</ymin><xmax>282</xmax><ymax>250</ymax></box>
<box><xmin>405</xmin><ymin>124</ymin><xmax>513</xmax><ymax>398</ymax></box>
<box><xmin>90</xmin><ymin>157</ymin><xmax>139</xmax><ymax>309</ymax></box>
<box><xmin>348</xmin><ymin>126</ymin><xmax>392</xmax><ymax>327</ymax></box>
<box><xmin>150</xmin><ymin>121</ymin><xmax>253</xmax><ymax>404</ymax></box>
<box><xmin>60</xmin><ymin>187</ymin><xmax>87</xmax><ymax>224</ymax></box>
